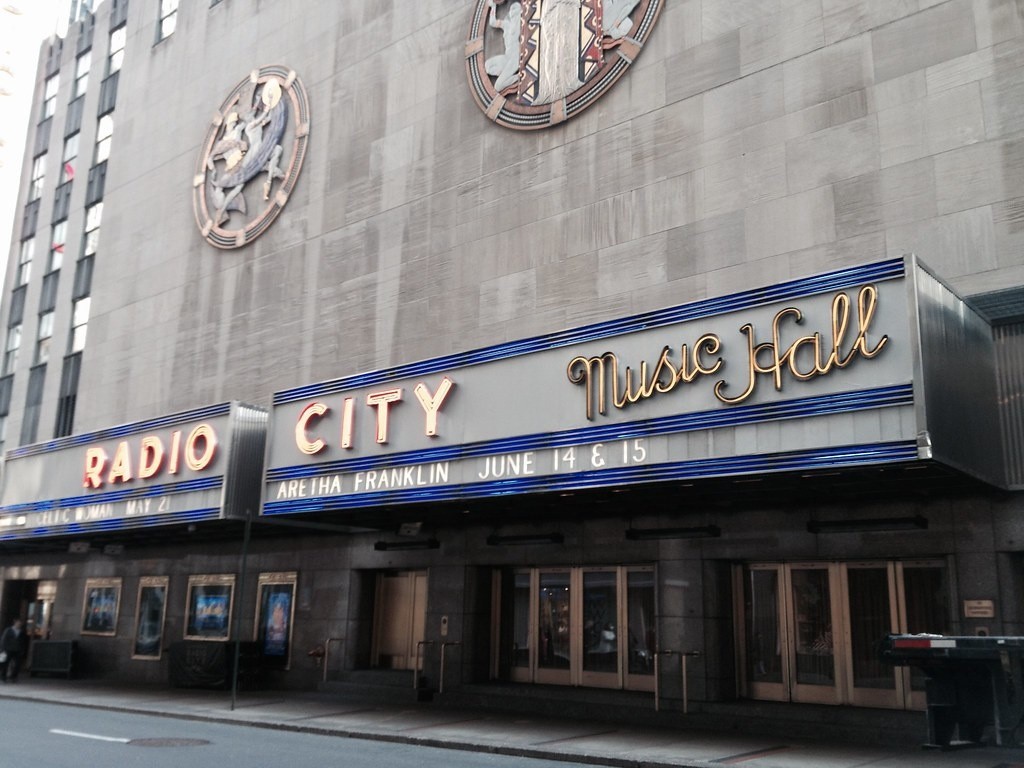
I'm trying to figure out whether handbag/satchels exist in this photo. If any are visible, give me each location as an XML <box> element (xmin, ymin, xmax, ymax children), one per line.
<box><xmin>0</xmin><ymin>651</ymin><xmax>7</xmax><ymax>663</ymax></box>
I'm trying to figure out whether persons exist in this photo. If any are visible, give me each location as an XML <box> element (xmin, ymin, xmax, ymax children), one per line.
<box><xmin>0</xmin><ymin>617</ymin><xmax>26</xmax><ymax>683</ymax></box>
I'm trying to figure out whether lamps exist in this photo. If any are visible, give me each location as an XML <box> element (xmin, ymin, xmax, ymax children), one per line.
<box><xmin>486</xmin><ymin>524</ymin><xmax>563</xmax><ymax>545</ymax></box>
<box><xmin>374</xmin><ymin>531</ymin><xmax>440</xmax><ymax>551</ymax></box>
<box><xmin>806</xmin><ymin>499</ymin><xmax>927</xmax><ymax>534</ymax></box>
<box><xmin>625</xmin><ymin>515</ymin><xmax>721</xmax><ymax>541</ymax></box>
